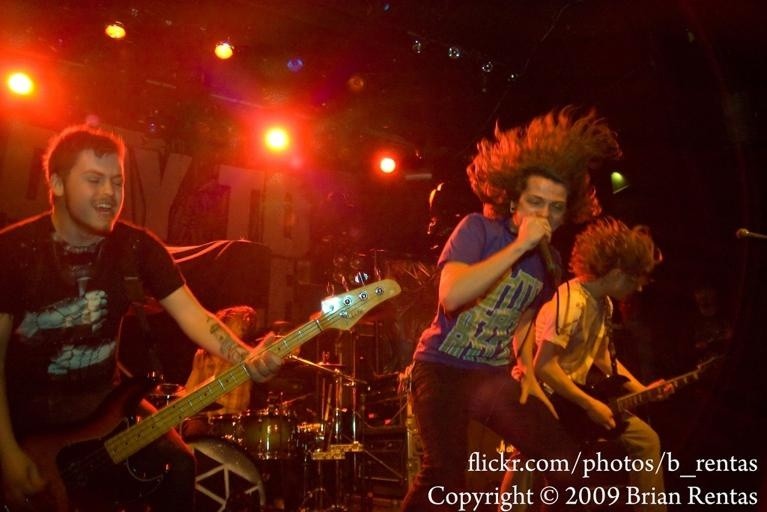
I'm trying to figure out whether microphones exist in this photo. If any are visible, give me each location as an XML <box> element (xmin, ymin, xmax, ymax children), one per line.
<box><xmin>539</xmin><ymin>235</ymin><xmax>560</xmax><ymax>282</ymax></box>
<box><xmin>736</xmin><ymin>228</ymin><xmax>767</xmax><ymax>243</ymax></box>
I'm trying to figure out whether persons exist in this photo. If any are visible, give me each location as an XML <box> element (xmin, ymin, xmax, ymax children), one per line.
<box><xmin>1</xmin><ymin>126</ymin><xmax>285</xmax><ymax>512</ymax></box>
<box><xmin>173</xmin><ymin>305</ymin><xmax>257</xmax><ymax>438</ymax></box>
<box><xmin>495</xmin><ymin>219</ymin><xmax>675</xmax><ymax>512</ymax></box>
<box><xmin>397</xmin><ymin>108</ymin><xmax>623</xmax><ymax>509</ymax></box>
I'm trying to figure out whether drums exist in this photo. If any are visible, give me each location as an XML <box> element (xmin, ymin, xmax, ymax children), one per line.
<box><xmin>234</xmin><ymin>410</ymin><xmax>298</xmax><ymax>460</ymax></box>
<box><xmin>181</xmin><ymin>435</ymin><xmax>265</xmax><ymax>512</ymax></box>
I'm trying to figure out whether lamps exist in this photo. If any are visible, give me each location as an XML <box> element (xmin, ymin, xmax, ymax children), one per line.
<box><xmin>106</xmin><ymin>16</ymin><xmax>128</xmax><ymax>39</ymax></box>
<box><xmin>212</xmin><ymin>34</ymin><xmax>236</xmax><ymax>61</ymax></box>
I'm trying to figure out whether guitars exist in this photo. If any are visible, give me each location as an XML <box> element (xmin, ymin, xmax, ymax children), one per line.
<box><xmin>552</xmin><ymin>356</ymin><xmax>723</xmax><ymax>445</ymax></box>
<box><xmin>1</xmin><ymin>267</ymin><xmax>401</xmax><ymax>511</ymax></box>
